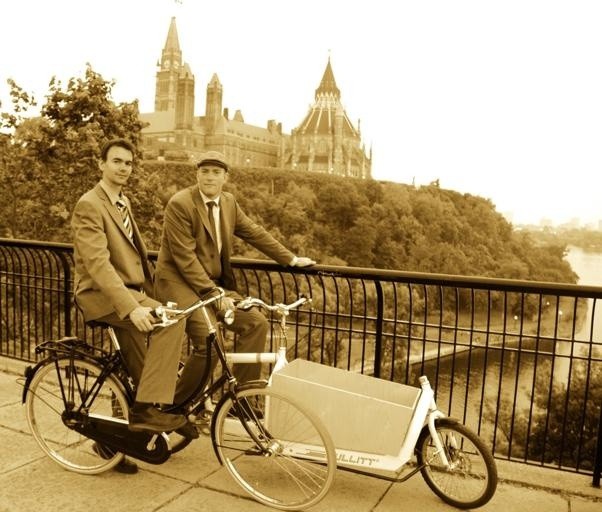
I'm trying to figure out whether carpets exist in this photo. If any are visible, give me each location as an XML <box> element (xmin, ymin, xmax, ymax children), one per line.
<box><xmin>203</xmin><ymin>201</ymin><xmax>219</xmax><ymax>254</ymax></box>
<box><xmin>116</xmin><ymin>202</ymin><xmax>134</xmax><ymax>243</ymax></box>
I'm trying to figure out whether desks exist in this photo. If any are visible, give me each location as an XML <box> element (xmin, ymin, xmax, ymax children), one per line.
<box><xmin>513</xmin><ymin>316</ymin><xmax>518</xmax><ymax>329</ymax></box>
<box><xmin>559</xmin><ymin>311</ymin><xmax>563</xmax><ymax>325</ymax></box>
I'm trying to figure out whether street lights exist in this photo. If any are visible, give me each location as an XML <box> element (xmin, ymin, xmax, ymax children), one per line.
<box><xmin>559</xmin><ymin>311</ymin><xmax>563</xmax><ymax>325</ymax></box>
<box><xmin>513</xmin><ymin>316</ymin><xmax>518</xmax><ymax>329</ymax></box>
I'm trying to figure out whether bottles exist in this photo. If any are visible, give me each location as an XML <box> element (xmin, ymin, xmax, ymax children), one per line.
<box><xmin>197</xmin><ymin>151</ymin><xmax>228</xmax><ymax>171</ymax></box>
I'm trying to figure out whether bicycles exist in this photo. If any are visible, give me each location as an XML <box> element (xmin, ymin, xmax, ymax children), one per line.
<box><xmin>16</xmin><ymin>286</ymin><xmax>339</xmax><ymax>511</ymax></box>
<box><xmin>155</xmin><ymin>291</ymin><xmax>498</xmax><ymax>512</ymax></box>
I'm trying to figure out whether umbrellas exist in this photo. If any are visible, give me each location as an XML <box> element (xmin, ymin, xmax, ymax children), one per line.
<box><xmin>161</xmin><ymin>408</ymin><xmax>199</xmax><ymax>439</ymax></box>
<box><xmin>92</xmin><ymin>442</ymin><xmax>138</xmax><ymax>474</ymax></box>
<box><xmin>225</xmin><ymin>395</ymin><xmax>265</xmax><ymax>420</ymax></box>
<box><xmin>128</xmin><ymin>405</ymin><xmax>187</xmax><ymax>431</ymax></box>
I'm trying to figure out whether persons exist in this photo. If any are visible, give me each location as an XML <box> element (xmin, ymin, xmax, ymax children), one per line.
<box><xmin>66</xmin><ymin>138</ymin><xmax>190</xmax><ymax>474</ymax></box>
<box><xmin>151</xmin><ymin>150</ymin><xmax>318</xmax><ymax>441</ymax></box>
